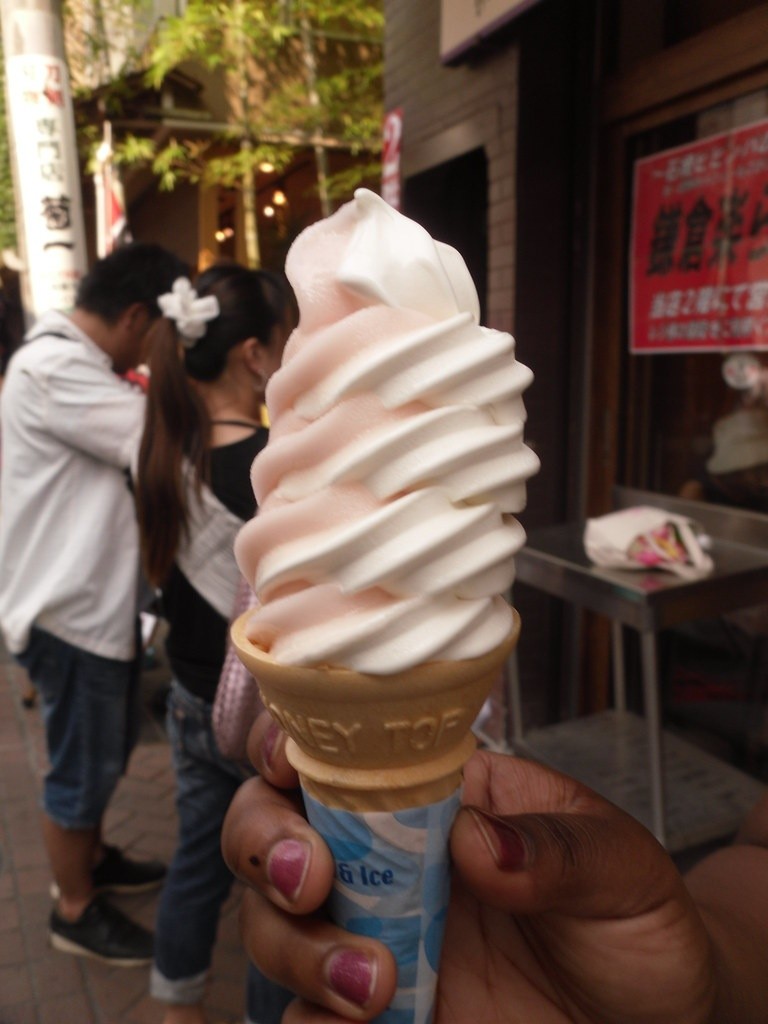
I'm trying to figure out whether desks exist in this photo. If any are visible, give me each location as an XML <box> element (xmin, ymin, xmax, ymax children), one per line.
<box><xmin>501</xmin><ymin>483</ymin><xmax>768</xmax><ymax>857</ymax></box>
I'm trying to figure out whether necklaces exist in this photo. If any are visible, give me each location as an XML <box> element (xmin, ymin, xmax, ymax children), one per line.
<box><xmin>210</xmin><ymin>419</ymin><xmax>260</xmax><ymax>432</ymax></box>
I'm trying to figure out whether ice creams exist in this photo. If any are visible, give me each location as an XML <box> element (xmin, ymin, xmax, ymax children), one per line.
<box><xmin>229</xmin><ymin>187</ymin><xmax>541</xmax><ymax>816</ymax></box>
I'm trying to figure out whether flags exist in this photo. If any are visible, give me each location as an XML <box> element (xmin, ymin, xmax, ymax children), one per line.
<box><xmin>99</xmin><ymin>152</ymin><xmax>130</xmax><ymax>253</ymax></box>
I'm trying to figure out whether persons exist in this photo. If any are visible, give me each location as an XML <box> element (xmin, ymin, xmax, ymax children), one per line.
<box><xmin>132</xmin><ymin>258</ymin><xmax>296</xmax><ymax>1024</ymax></box>
<box><xmin>675</xmin><ymin>405</ymin><xmax>768</xmax><ymax>514</ymax></box>
<box><xmin>0</xmin><ymin>245</ymin><xmax>195</xmax><ymax>968</ymax></box>
<box><xmin>220</xmin><ymin>702</ymin><xmax>768</xmax><ymax>1024</ymax></box>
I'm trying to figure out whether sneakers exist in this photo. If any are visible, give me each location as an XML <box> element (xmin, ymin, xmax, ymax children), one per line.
<box><xmin>50</xmin><ymin>893</ymin><xmax>154</xmax><ymax>966</ymax></box>
<box><xmin>49</xmin><ymin>844</ymin><xmax>168</xmax><ymax>897</ymax></box>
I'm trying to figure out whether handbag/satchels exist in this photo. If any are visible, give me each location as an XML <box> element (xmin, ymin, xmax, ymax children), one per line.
<box><xmin>584</xmin><ymin>506</ymin><xmax>715</xmax><ymax>580</ymax></box>
